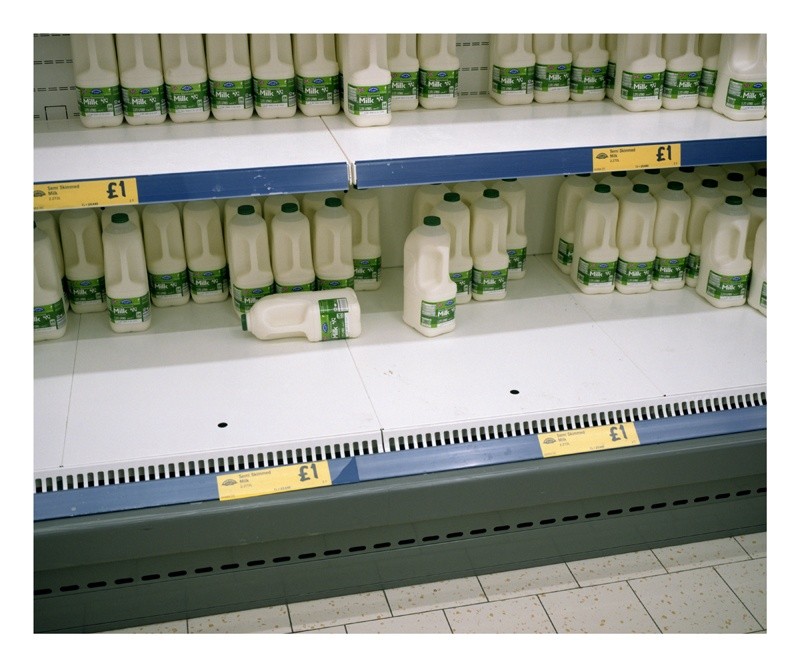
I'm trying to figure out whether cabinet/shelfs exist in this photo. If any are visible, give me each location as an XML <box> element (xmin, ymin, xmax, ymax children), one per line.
<box><xmin>33</xmin><ymin>93</ymin><xmax>768</xmax><ymax>635</ymax></box>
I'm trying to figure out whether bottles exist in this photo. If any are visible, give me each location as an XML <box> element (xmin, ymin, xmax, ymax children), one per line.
<box><xmin>487</xmin><ymin>34</ymin><xmax>767</xmax><ymax>122</ymax></box>
<box><xmin>552</xmin><ymin>162</ymin><xmax>767</xmax><ymax>318</ymax></box>
<box><xmin>34</xmin><ymin>187</ymin><xmax>381</xmax><ymax>343</ymax></box>
<box><xmin>403</xmin><ymin>178</ymin><xmax>527</xmax><ymax>338</ymax></box>
<box><xmin>70</xmin><ymin>35</ymin><xmax>461</xmax><ymax>128</ymax></box>
